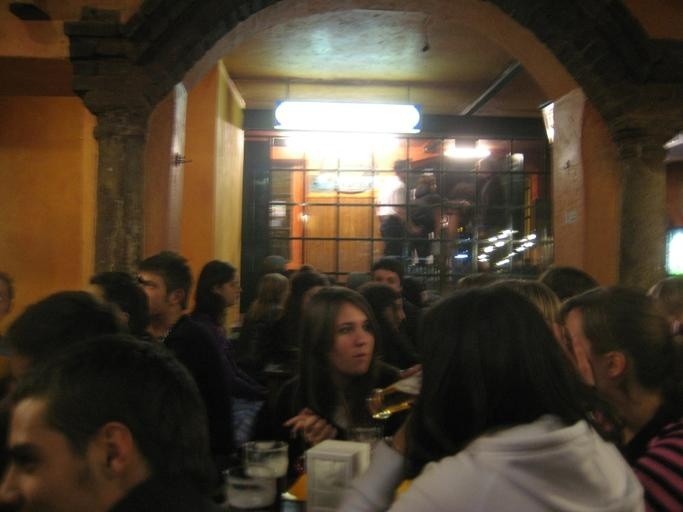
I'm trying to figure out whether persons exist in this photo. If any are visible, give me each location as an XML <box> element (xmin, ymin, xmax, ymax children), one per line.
<box><xmin>232</xmin><ymin>254</ymin><xmax>426</xmax><ymax>479</ymax></box>
<box><xmin>373</xmin><ymin>139</ymin><xmax>524</xmax><ymax>278</ymax></box>
<box><xmin>1</xmin><ymin>250</ymin><xmax>243</xmax><ymax>511</ymax></box>
<box><xmin>338</xmin><ymin>258</ymin><xmax>682</xmax><ymax>511</ymax></box>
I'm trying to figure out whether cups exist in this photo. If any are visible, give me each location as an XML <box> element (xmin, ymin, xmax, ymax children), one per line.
<box><xmin>224</xmin><ymin>464</ymin><xmax>278</xmax><ymax>512</ymax></box>
<box><xmin>243</xmin><ymin>440</ymin><xmax>289</xmax><ymax>491</ymax></box>
